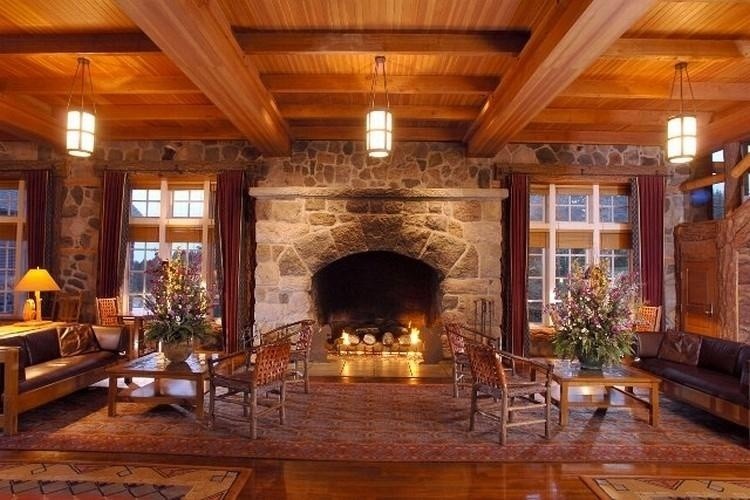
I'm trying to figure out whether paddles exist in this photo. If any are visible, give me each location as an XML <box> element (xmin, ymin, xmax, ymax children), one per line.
<box><xmin>0</xmin><ymin>455</ymin><xmax>253</xmax><ymax>499</ymax></box>
<box><xmin>578</xmin><ymin>471</ymin><xmax>750</xmax><ymax>499</ymax></box>
<box><xmin>0</xmin><ymin>374</ymin><xmax>750</xmax><ymax>464</ymax></box>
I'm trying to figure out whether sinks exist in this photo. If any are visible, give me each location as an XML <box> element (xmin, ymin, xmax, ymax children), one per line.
<box><xmin>0</xmin><ymin>322</ymin><xmax>130</xmax><ymax>435</ymax></box>
<box><xmin>625</xmin><ymin>327</ymin><xmax>750</xmax><ymax>432</ymax></box>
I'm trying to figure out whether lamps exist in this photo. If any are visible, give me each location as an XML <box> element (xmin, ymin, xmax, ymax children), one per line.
<box><xmin>444</xmin><ymin>322</ymin><xmax>553</xmax><ymax>445</ymax></box>
<box><xmin>94</xmin><ymin>296</ymin><xmax>134</xmax><ymax>327</ymax></box>
<box><xmin>51</xmin><ymin>291</ymin><xmax>84</xmax><ymax>323</ymax></box>
<box><xmin>207</xmin><ymin>318</ymin><xmax>316</xmax><ymax>440</ymax></box>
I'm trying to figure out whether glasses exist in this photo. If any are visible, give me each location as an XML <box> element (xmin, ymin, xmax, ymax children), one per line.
<box><xmin>15</xmin><ymin>266</ymin><xmax>61</xmax><ymax>326</ymax></box>
<box><xmin>363</xmin><ymin>57</ymin><xmax>395</xmax><ymax>159</ymax></box>
<box><xmin>64</xmin><ymin>56</ymin><xmax>97</xmax><ymax>160</ymax></box>
<box><xmin>665</xmin><ymin>62</ymin><xmax>699</xmax><ymax>165</ymax></box>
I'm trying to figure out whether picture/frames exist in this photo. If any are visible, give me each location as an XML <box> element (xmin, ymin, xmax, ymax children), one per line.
<box><xmin>108</xmin><ymin>308</ymin><xmax>156</xmax><ymax>358</ymax></box>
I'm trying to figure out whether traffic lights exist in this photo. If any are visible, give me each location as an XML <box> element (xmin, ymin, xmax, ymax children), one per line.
<box><xmin>56</xmin><ymin>323</ymin><xmax>103</xmax><ymax>357</ymax></box>
<box><xmin>658</xmin><ymin>329</ymin><xmax>703</xmax><ymax>367</ymax></box>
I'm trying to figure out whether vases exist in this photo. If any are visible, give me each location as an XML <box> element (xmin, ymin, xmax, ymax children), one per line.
<box><xmin>141</xmin><ymin>249</ymin><xmax>210</xmax><ymax>343</ymax></box>
<box><xmin>543</xmin><ymin>259</ymin><xmax>641</xmax><ymax>367</ymax></box>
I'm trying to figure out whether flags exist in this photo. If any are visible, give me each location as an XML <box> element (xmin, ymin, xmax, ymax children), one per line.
<box><xmin>575</xmin><ymin>337</ymin><xmax>610</xmax><ymax>371</ymax></box>
<box><xmin>162</xmin><ymin>336</ymin><xmax>199</xmax><ymax>363</ymax></box>
